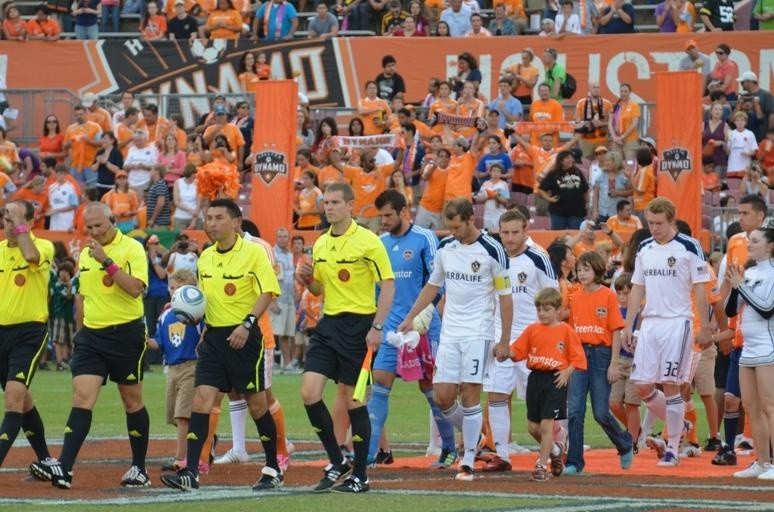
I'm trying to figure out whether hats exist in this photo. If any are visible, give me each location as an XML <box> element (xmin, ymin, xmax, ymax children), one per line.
<box><xmin>82</xmin><ymin>93</ymin><xmax>97</xmax><ymax>107</ymax></box>
<box><xmin>215</xmin><ymin>106</ymin><xmax>226</xmax><ymax>115</ymax></box>
<box><xmin>737</xmin><ymin>72</ymin><xmax>758</xmax><ymax>82</ymax></box>
<box><xmin>455</xmin><ymin>135</ymin><xmax>469</xmax><ymax>150</ymax></box>
<box><xmin>638</xmin><ymin>136</ymin><xmax>655</xmax><ymax>151</ymax></box>
<box><xmin>116</xmin><ymin>170</ymin><xmax>128</xmax><ymax>178</ymax></box>
<box><xmin>173</xmin><ymin>0</ymin><xmax>186</xmax><ymax>6</ymax></box>
<box><xmin>595</xmin><ymin>146</ymin><xmax>608</xmax><ymax>154</ymax></box>
<box><xmin>707</xmin><ymin>80</ymin><xmax>723</xmax><ymax>88</ymax></box>
<box><xmin>684</xmin><ymin>40</ymin><xmax>696</xmax><ymax>50</ymax></box>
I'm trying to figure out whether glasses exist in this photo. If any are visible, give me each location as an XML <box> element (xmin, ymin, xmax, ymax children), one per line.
<box><xmin>47</xmin><ymin>120</ymin><xmax>57</xmax><ymax>123</ymax></box>
<box><xmin>241</xmin><ymin>107</ymin><xmax>250</xmax><ymax>109</ymax></box>
<box><xmin>716</xmin><ymin>51</ymin><xmax>725</xmax><ymax>55</ymax></box>
<box><xmin>134</xmin><ymin>130</ymin><xmax>145</xmax><ymax>134</ymax></box>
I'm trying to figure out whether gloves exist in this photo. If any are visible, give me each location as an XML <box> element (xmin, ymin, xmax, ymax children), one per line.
<box><xmin>410</xmin><ymin>304</ymin><xmax>435</xmax><ymax>335</ymax></box>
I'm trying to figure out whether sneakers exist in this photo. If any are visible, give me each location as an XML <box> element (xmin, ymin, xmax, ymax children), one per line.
<box><xmin>313</xmin><ymin>444</ymin><xmax>393</xmax><ymax>493</ymax></box>
<box><xmin>161</xmin><ymin>434</ymin><xmax>250</xmax><ymax>493</ymax></box>
<box><xmin>534</xmin><ymin>428</ymin><xmax>591</xmax><ymax>482</ymax></box>
<box><xmin>249</xmin><ymin>439</ymin><xmax>295</xmax><ymax>490</ymax></box>
<box><xmin>619</xmin><ymin>427</ymin><xmax>774</xmax><ymax>481</ymax></box>
<box><xmin>22</xmin><ymin>458</ymin><xmax>73</xmax><ymax>490</ymax></box>
<box><xmin>120</xmin><ymin>466</ymin><xmax>151</xmax><ymax>488</ymax></box>
<box><xmin>426</xmin><ymin>427</ymin><xmax>533</xmax><ymax>481</ymax></box>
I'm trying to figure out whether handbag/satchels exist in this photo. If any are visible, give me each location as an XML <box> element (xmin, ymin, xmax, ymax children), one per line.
<box><xmin>561</xmin><ymin>73</ymin><xmax>576</xmax><ymax>98</ymax></box>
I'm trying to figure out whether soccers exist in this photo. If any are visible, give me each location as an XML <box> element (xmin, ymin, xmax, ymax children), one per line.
<box><xmin>170</xmin><ymin>285</ymin><xmax>205</xmax><ymax>322</ymax></box>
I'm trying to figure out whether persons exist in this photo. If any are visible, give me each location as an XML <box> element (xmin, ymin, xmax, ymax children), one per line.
<box><xmin>0</xmin><ymin>0</ymin><xmax>773</xmax><ymax>494</ymax></box>
